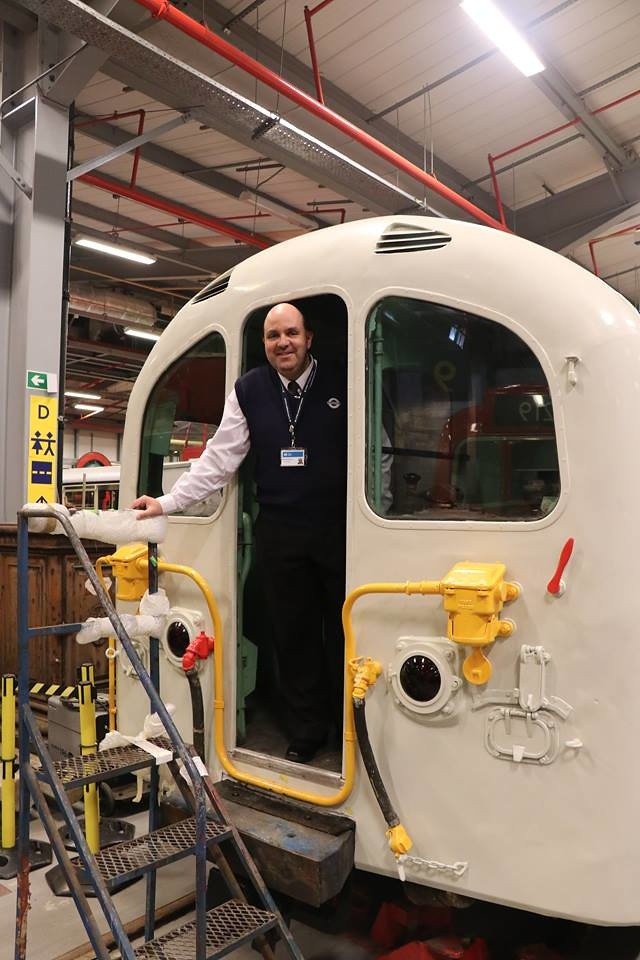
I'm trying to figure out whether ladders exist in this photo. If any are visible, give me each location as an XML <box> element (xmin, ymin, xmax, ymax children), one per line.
<box><xmin>13</xmin><ymin>504</ymin><xmax>307</xmax><ymax>960</ymax></box>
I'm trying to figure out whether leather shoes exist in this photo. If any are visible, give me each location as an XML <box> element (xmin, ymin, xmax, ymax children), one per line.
<box><xmin>287</xmin><ymin>726</ymin><xmax>328</xmax><ymax>763</ymax></box>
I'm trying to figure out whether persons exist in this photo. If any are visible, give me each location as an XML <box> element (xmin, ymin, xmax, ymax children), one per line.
<box><xmin>298</xmin><ymin>458</ymin><xmax>303</xmax><ymax>464</ymax></box>
<box><xmin>129</xmin><ymin>302</ymin><xmax>392</xmax><ymax>762</ymax></box>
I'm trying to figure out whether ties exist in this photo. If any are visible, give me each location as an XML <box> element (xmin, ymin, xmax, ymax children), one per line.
<box><xmin>286</xmin><ymin>382</ymin><xmax>300</xmax><ymax>425</ymax></box>
